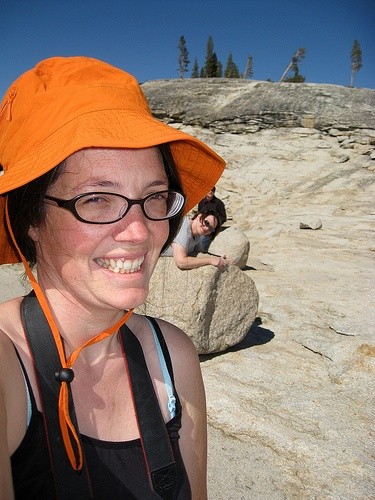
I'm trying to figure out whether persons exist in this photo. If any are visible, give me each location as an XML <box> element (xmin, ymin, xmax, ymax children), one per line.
<box><xmin>160</xmin><ymin>185</ymin><xmax>228</xmax><ymax>273</ymax></box>
<box><xmin>0</xmin><ymin>57</ymin><xmax>227</xmax><ymax>500</ymax></box>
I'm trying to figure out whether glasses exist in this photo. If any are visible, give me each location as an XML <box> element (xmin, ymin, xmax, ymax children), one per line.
<box><xmin>44</xmin><ymin>189</ymin><xmax>186</xmax><ymax>224</ymax></box>
<box><xmin>204</xmin><ymin>220</ymin><xmax>215</xmax><ymax>232</ymax></box>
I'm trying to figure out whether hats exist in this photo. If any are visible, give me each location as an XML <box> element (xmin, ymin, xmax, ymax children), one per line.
<box><xmin>0</xmin><ymin>56</ymin><xmax>224</xmax><ymax>265</ymax></box>
